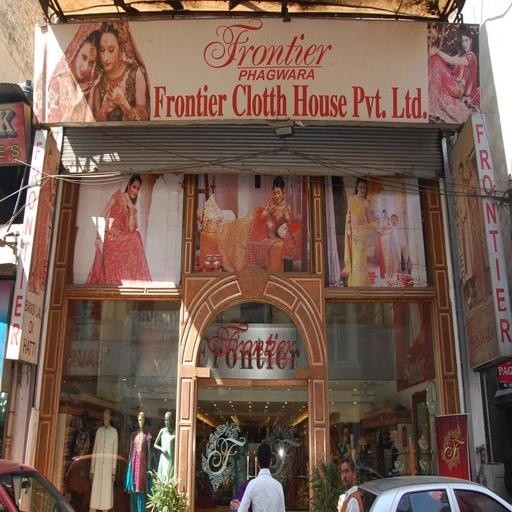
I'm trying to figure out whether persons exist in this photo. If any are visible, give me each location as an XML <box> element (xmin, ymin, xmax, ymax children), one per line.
<box><xmin>47</xmin><ymin>21</ymin><xmax>152</xmax><ymax>121</ymax></box>
<box><xmin>429</xmin><ymin>27</ymin><xmax>480</xmax><ymax>123</ymax></box>
<box><xmin>338</xmin><ymin>178</ymin><xmax>407</xmax><ymax>285</ymax></box>
<box><xmin>152</xmin><ymin>412</ymin><xmax>176</xmax><ymax>479</ymax></box>
<box><xmin>83</xmin><ymin>178</ymin><xmax>152</xmax><ymax>283</ymax></box>
<box><xmin>237</xmin><ymin>444</ymin><xmax>287</xmax><ymax>512</ymax></box>
<box><xmin>87</xmin><ymin>408</ymin><xmax>118</xmax><ymax>512</ymax></box>
<box><xmin>337</xmin><ymin>458</ymin><xmax>363</xmax><ymax>512</ymax></box>
<box><xmin>125</xmin><ymin>412</ymin><xmax>153</xmax><ymax>512</ymax></box>
<box><xmin>452</xmin><ymin>158</ymin><xmax>488</xmax><ymax>305</ymax></box>
<box><xmin>219</xmin><ymin>176</ymin><xmax>297</xmax><ymax>271</ymax></box>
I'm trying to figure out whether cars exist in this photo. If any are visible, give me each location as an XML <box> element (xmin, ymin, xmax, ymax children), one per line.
<box><xmin>342</xmin><ymin>476</ymin><xmax>512</xmax><ymax>512</ymax></box>
<box><xmin>0</xmin><ymin>458</ymin><xmax>71</xmax><ymax>512</ymax></box>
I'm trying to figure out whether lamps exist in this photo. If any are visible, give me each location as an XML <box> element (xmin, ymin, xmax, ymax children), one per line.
<box><xmin>272</xmin><ymin>120</ymin><xmax>294</xmax><ymax>139</ymax></box>
